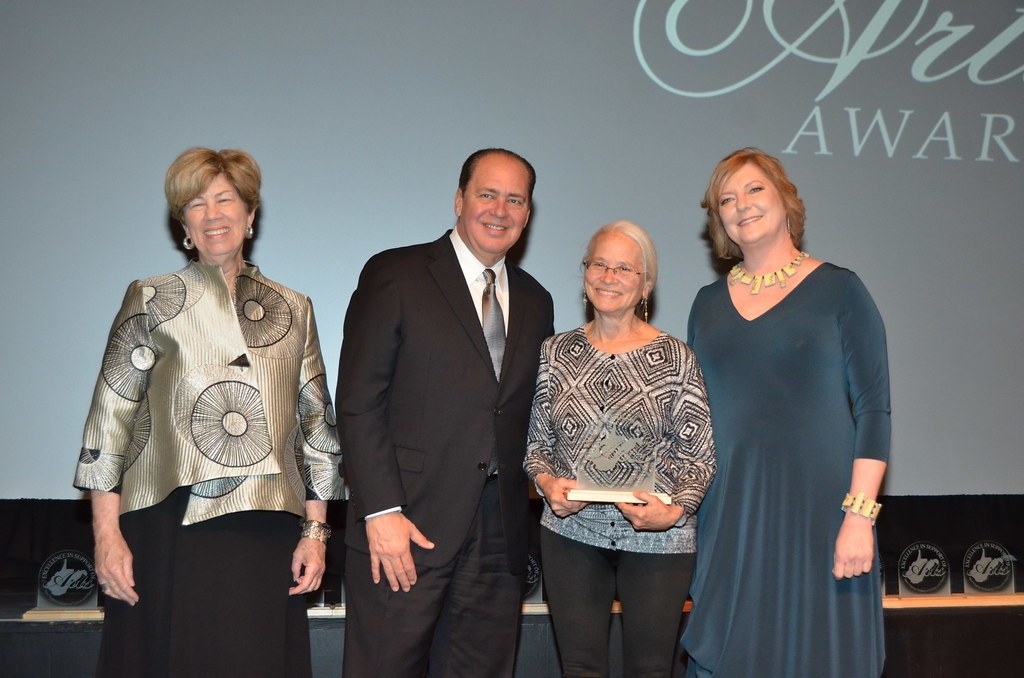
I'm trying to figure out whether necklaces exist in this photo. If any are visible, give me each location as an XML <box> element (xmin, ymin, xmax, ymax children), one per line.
<box><xmin>730</xmin><ymin>251</ymin><xmax>809</xmax><ymax>295</ymax></box>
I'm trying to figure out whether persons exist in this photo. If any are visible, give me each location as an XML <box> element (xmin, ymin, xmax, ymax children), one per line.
<box><xmin>524</xmin><ymin>219</ymin><xmax>718</xmax><ymax>678</ymax></box>
<box><xmin>335</xmin><ymin>147</ymin><xmax>555</xmax><ymax>678</ymax></box>
<box><xmin>73</xmin><ymin>148</ymin><xmax>347</xmax><ymax>678</ymax></box>
<box><xmin>680</xmin><ymin>147</ymin><xmax>892</xmax><ymax>678</ymax></box>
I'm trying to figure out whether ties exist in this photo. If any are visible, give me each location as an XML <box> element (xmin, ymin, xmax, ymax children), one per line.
<box><xmin>482</xmin><ymin>269</ymin><xmax>506</xmax><ymax>383</ymax></box>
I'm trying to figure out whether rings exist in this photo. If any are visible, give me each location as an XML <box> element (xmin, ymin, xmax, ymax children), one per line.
<box><xmin>99</xmin><ymin>583</ymin><xmax>109</xmax><ymax>591</ymax></box>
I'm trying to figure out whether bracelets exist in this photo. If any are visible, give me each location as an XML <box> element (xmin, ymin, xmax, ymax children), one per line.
<box><xmin>842</xmin><ymin>492</ymin><xmax>882</xmax><ymax>526</ymax></box>
<box><xmin>301</xmin><ymin>520</ymin><xmax>331</xmax><ymax>545</ymax></box>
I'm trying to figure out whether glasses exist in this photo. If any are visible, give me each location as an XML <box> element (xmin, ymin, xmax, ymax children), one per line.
<box><xmin>583</xmin><ymin>259</ymin><xmax>648</xmax><ymax>280</ymax></box>
